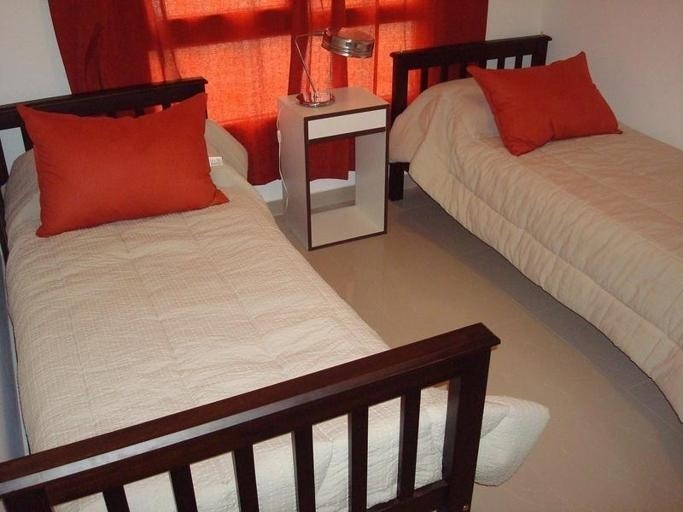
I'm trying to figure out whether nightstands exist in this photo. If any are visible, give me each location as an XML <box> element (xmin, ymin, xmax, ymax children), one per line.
<box><xmin>276</xmin><ymin>88</ymin><xmax>394</xmax><ymax>252</ymax></box>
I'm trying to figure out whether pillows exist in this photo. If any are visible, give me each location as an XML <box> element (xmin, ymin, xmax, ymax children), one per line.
<box><xmin>467</xmin><ymin>52</ymin><xmax>622</xmax><ymax>155</ymax></box>
<box><xmin>15</xmin><ymin>92</ymin><xmax>228</xmax><ymax>237</ymax></box>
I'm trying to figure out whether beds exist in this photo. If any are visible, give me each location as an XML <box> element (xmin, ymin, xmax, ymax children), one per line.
<box><xmin>389</xmin><ymin>35</ymin><xmax>682</xmax><ymax>417</ymax></box>
<box><xmin>0</xmin><ymin>78</ymin><xmax>499</xmax><ymax>510</ymax></box>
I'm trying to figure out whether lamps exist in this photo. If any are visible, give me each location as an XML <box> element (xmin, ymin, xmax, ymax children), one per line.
<box><xmin>292</xmin><ymin>28</ymin><xmax>372</xmax><ymax>109</ymax></box>
